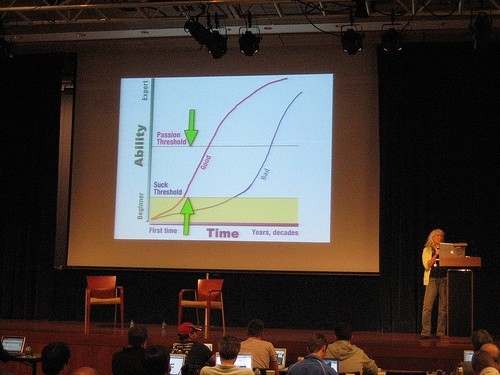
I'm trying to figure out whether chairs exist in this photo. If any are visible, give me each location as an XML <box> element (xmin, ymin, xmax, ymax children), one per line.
<box><xmin>178</xmin><ymin>277</ymin><xmax>226</xmax><ymax>339</ymax></box>
<box><xmin>83</xmin><ymin>275</ymin><xmax>126</xmax><ymax>333</ymax></box>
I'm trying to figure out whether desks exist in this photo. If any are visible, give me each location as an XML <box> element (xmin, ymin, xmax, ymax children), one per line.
<box><xmin>13</xmin><ymin>354</ymin><xmax>43</xmax><ymax>375</ymax></box>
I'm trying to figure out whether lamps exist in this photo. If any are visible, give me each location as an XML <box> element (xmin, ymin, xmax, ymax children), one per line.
<box><xmin>467</xmin><ymin>7</ymin><xmax>492</xmax><ymax>50</ymax></box>
<box><xmin>184</xmin><ymin>8</ymin><xmax>263</xmax><ymax>60</ymax></box>
<box><xmin>340</xmin><ymin>13</ymin><xmax>369</xmax><ymax>55</ymax></box>
<box><xmin>381</xmin><ymin>13</ymin><xmax>410</xmax><ymax>53</ymax></box>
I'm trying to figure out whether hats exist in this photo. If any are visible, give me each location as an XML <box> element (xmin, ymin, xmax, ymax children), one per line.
<box><xmin>176</xmin><ymin>322</ymin><xmax>202</xmax><ymax>336</ymax></box>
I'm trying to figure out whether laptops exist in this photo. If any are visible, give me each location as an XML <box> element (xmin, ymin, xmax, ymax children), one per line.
<box><xmin>275</xmin><ymin>347</ymin><xmax>286</xmax><ymax>369</ymax></box>
<box><xmin>439</xmin><ymin>243</ymin><xmax>466</xmax><ymax>258</ymax></box>
<box><xmin>1</xmin><ymin>335</ymin><xmax>25</xmax><ymax>355</ymax></box>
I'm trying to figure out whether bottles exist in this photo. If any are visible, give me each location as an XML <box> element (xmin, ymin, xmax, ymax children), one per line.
<box><xmin>130</xmin><ymin>320</ymin><xmax>135</xmax><ymax>329</ymax></box>
<box><xmin>162</xmin><ymin>321</ymin><xmax>167</xmax><ymax>336</ymax></box>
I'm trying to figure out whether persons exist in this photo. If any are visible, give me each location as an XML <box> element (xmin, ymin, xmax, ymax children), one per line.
<box><xmin>472</xmin><ymin>351</ymin><xmax>500</xmax><ymax>375</ymax></box>
<box><xmin>233</xmin><ymin>319</ymin><xmax>281</xmax><ymax>375</ymax></box>
<box><xmin>171</xmin><ymin>322</ymin><xmax>216</xmax><ymax>375</ymax></box>
<box><xmin>310</xmin><ymin>322</ymin><xmax>388</xmax><ymax>375</ymax></box>
<box><xmin>418</xmin><ymin>228</ymin><xmax>453</xmax><ymax>338</ymax></box>
<box><xmin>110</xmin><ymin>328</ymin><xmax>152</xmax><ymax>375</ymax></box>
<box><xmin>0</xmin><ymin>336</ymin><xmax>12</xmax><ymax>375</ymax></box>
<box><xmin>41</xmin><ymin>342</ymin><xmax>71</xmax><ymax>375</ymax></box>
<box><xmin>463</xmin><ymin>328</ymin><xmax>499</xmax><ymax>375</ymax></box>
<box><xmin>199</xmin><ymin>335</ymin><xmax>256</xmax><ymax>375</ymax></box>
<box><xmin>479</xmin><ymin>343</ymin><xmax>500</xmax><ymax>365</ymax></box>
<box><xmin>286</xmin><ymin>333</ymin><xmax>340</xmax><ymax>375</ymax></box>
<box><xmin>143</xmin><ymin>345</ymin><xmax>172</xmax><ymax>375</ymax></box>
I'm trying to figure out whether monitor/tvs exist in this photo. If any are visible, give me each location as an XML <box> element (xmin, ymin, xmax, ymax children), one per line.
<box><xmin>215</xmin><ymin>352</ymin><xmax>253</xmax><ymax>370</ymax></box>
<box><xmin>167</xmin><ymin>354</ymin><xmax>185</xmax><ymax>375</ymax></box>
<box><xmin>323</xmin><ymin>357</ymin><xmax>339</xmax><ymax>373</ymax></box>
<box><xmin>464</xmin><ymin>350</ymin><xmax>474</xmax><ymax>361</ymax></box>
